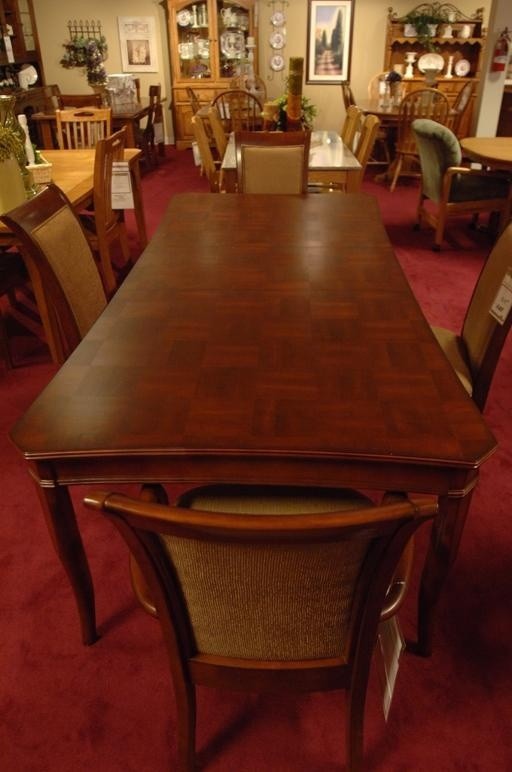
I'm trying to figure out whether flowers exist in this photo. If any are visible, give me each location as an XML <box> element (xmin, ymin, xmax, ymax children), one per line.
<box><xmin>56</xmin><ymin>35</ymin><xmax>110</xmax><ymax>85</ymax></box>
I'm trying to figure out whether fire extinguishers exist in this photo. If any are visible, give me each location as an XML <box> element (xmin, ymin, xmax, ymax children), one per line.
<box><xmin>491</xmin><ymin>27</ymin><xmax>512</xmax><ymax>71</ymax></box>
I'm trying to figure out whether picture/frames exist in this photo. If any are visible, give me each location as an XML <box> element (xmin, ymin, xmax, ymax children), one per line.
<box><xmin>305</xmin><ymin>1</ymin><xmax>354</xmax><ymax>85</ymax></box>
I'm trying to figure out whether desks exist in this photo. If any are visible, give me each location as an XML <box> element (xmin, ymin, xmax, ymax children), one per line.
<box><xmin>459</xmin><ymin>137</ymin><xmax>512</xmax><ymax>170</ymax></box>
<box><xmin>8</xmin><ymin>195</ymin><xmax>499</xmax><ymax>656</ymax></box>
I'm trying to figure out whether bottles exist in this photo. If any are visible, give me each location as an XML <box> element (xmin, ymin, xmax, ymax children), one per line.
<box><xmin>18</xmin><ymin>115</ymin><xmax>42</xmax><ymax>167</ymax></box>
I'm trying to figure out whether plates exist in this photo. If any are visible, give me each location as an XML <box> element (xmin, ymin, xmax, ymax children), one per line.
<box><xmin>177</xmin><ymin>8</ymin><xmax>193</xmax><ymax>26</ymax></box>
<box><xmin>454</xmin><ymin>58</ymin><xmax>475</xmax><ymax>77</ymax></box>
<box><xmin>220</xmin><ymin>31</ymin><xmax>248</xmax><ymax>57</ymax></box>
<box><xmin>271</xmin><ymin>11</ymin><xmax>285</xmax><ymax>71</ymax></box>
<box><xmin>22</xmin><ymin>64</ymin><xmax>38</xmax><ymax>85</ymax></box>
<box><xmin>419</xmin><ymin>53</ymin><xmax>445</xmax><ymax>75</ymax></box>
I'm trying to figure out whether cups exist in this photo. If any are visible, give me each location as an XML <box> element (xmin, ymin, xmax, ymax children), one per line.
<box><xmin>446</xmin><ymin>11</ymin><xmax>457</xmax><ymax>24</ymax></box>
<box><xmin>394</xmin><ymin>62</ymin><xmax>405</xmax><ymax>74</ymax></box>
<box><xmin>380</xmin><ymin>80</ymin><xmax>409</xmax><ymax>106</ymax></box>
<box><xmin>18</xmin><ymin>71</ymin><xmax>28</xmax><ymax>91</ymax></box>
<box><xmin>220</xmin><ymin>5</ymin><xmax>249</xmax><ymax>29</ymax></box>
<box><xmin>177</xmin><ymin>40</ymin><xmax>210</xmax><ymax>58</ymax></box>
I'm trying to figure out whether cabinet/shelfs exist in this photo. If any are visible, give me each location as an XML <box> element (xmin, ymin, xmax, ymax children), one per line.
<box><xmin>381</xmin><ymin>2</ymin><xmax>486</xmax><ymax>140</ymax></box>
<box><xmin>159</xmin><ymin>0</ymin><xmax>263</xmax><ymax>152</ymax></box>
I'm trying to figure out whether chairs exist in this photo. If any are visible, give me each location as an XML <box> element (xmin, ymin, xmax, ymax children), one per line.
<box><xmin>232</xmin><ymin>130</ymin><xmax>312</xmax><ymax>195</ymax></box>
<box><xmin>81</xmin><ymin>483</ymin><xmax>441</xmax><ymax>772</ymax></box>
<box><xmin>1</xmin><ymin>106</ymin><xmax>148</xmax><ymax>363</ymax></box>
<box><xmin>186</xmin><ymin>76</ymin><xmax>485</xmax><ymax>189</ymax></box>
<box><xmin>31</xmin><ymin>76</ymin><xmax>167</xmax><ymax>179</ymax></box>
<box><xmin>412</xmin><ymin>120</ymin><xmax>512</xmax><ymax>254</ymax></box>
<box><xmin>430</xmin><ymin>225</ymin><xmax>512</xmax><ymax>414</ymax></box>
